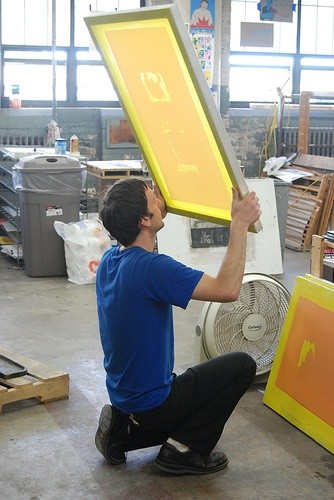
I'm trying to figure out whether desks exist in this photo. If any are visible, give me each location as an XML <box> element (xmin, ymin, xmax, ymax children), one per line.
<box><xmin>0</xmin><ymin>145</ymin><xmax>87</xmax><ymax>270</ymax></box>
<box><xmin>86</xmin><ymin>159</ymin><xmax>153</xmax><ymax>180</ymax></box>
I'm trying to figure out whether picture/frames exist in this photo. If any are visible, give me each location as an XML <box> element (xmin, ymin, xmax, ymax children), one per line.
<box><xmin>84</xmin><ymin>2</ymin><xmax>263</xmax><ymax>234</ymax></box>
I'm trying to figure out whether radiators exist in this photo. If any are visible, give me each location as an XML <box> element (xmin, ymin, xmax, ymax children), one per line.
<box><xmin>270</xmin><ymin>127</ymin><xmax>334</xmax><ymax>157</ymax></box>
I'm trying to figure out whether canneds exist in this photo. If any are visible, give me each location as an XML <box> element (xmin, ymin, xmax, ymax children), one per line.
<box><xmin>54</xmin><ymin>138</ymin><xmax>67</xmax><ymax>155</ymax></box>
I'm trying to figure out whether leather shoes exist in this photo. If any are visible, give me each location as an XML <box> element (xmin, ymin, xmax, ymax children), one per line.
<box><xmin>154</xmin><ymin>442</ymin><xmax>229</xmax><ymax>476</ymax></box>
<box><xmin>95</xmin><ymin>404</ymin><xmax>126</xmax><ymax>465</ymax></box>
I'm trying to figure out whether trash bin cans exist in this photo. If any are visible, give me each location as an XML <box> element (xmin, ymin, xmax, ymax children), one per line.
<box><xmin>12</xmin><ymin>154</ymin><xmax>87</xmax><ymax>277</ymax></box>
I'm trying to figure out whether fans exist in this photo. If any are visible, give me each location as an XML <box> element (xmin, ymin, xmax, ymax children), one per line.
<box><xmin>190</xmin><ymin>271</ymin><xmax>291</xmax><ymax>385</ymax></box>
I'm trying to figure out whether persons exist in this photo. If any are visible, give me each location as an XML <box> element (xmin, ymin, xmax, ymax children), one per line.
<box><xmin>95</xmin><ymin>178</ymin><xmax>264</xmax><ymax>474</ymax></box>
